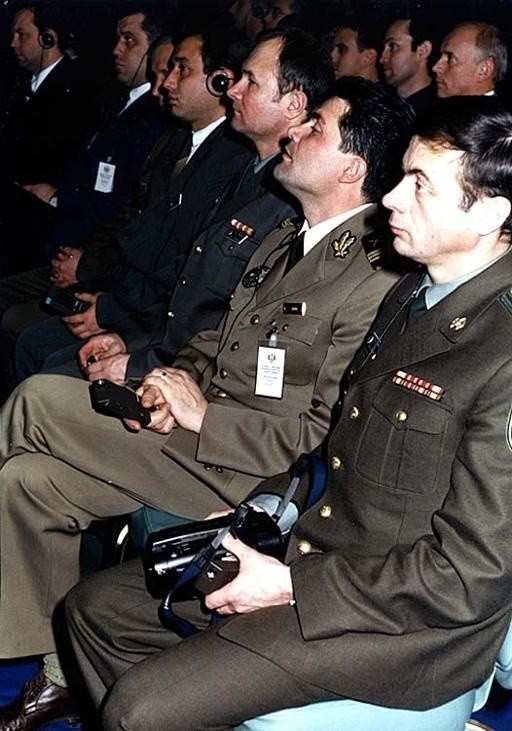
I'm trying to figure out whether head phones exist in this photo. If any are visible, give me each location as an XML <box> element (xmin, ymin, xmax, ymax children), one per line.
<box><xmin>212</xmin><ymin>72</ymin><xmax>230</xmax><ymax>93</ymax></box>
<box><xmin>42</xmin><ymin>31</ymin><xmax>56</xmax><ymax>48</ymax></box>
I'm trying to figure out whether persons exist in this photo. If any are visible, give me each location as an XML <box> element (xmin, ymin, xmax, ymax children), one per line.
<box><xmin>2</xmin><ymin>1</ymin><xmax>511</xmax><ymax>730</ymax></box>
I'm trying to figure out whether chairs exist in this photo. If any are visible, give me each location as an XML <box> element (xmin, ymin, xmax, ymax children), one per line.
<box><xmin>236</xmin><ymin>612</ymin><xmax>512</xmax><ymax>731</ymax></box>
<box><xmin>132</xmin><ymin>504</ymin><xmax>235</xmax><ymax>553</ymax></box>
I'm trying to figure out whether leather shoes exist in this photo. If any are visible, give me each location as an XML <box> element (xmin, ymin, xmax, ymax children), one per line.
<box><xmin>0</xmin><ymin>668</ymin><xmax>87</xmax><ymax>731</ymax></box>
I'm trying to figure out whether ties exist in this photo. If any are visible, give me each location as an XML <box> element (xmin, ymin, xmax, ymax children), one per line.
<box><xmin>238</xmin><ymin>164</ymin><xmax>257</xmax><ymax>195</ymax></box>
<box><xmin>404</xmin><ymin>284</ymin><xmax>432</xmax><ymax>334</ymax></box>
<box><xmin>282</xmin><ymin>232</ymin><xmax>307</xmax><ymax>278</ymax></box>
<box><xmin>160</xmin><ymin>133</ymin><xmax>194</xmax><ymax>197</ymax></box>
